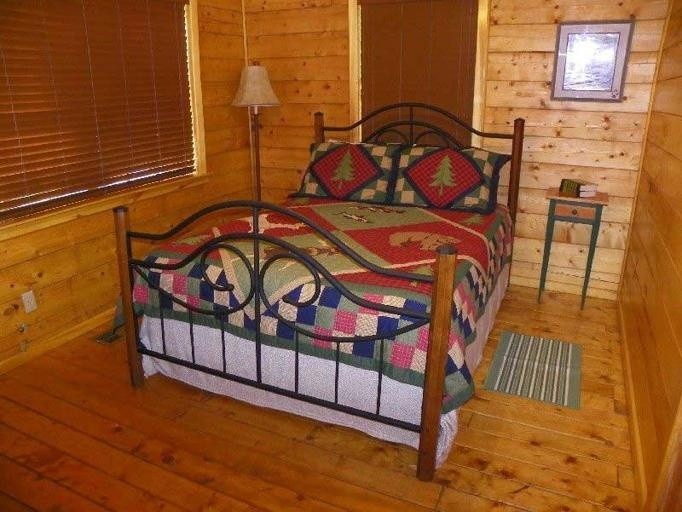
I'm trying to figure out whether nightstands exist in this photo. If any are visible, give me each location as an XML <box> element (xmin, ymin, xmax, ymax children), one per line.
<box><xmin>536</xmin><ymin>187</ymin><xmax>610</xmax><ymax>310</ymax></box>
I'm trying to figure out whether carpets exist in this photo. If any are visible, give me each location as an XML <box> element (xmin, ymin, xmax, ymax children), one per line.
<box><xmin>482</xmin><ymin>328</ymin><xmax>582</xmax><ymax>411</ymax></box>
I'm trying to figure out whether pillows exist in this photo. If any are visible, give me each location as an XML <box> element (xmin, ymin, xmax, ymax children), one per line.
<box><xmin>288</xmin><ymin>139</ymin><xmax>512</xmax><ymax>216</ymax></box>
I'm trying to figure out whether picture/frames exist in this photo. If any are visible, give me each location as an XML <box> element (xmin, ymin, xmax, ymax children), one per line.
<box><xmin>549</xmin><ymin>19</ymin><xmax>635</xmax><ymax>103</ymax></box>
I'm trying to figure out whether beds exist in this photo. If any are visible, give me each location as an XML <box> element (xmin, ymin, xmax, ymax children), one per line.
<box><xmin>111</xmin><ymin>102</ymin><xmax>526</xmax><ymax>482</ymax></box>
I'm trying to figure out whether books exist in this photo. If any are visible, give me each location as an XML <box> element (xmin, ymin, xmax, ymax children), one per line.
<box><xmin>561</xmin><ymin>178</ymin><xmax>598</xmax><ymax>192</ymax></box>
<box><xmin>559</xmin><ymin>186</ymin><xmax>597</xmax><ymax>198</ymax></box>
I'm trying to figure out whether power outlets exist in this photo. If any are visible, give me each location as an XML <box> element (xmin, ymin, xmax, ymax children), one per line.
<box><xmin>20</xmin><ymin>290</ymin><xmax>37</xmax><ymax>314</ymax></box>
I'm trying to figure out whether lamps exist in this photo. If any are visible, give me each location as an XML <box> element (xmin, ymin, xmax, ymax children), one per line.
<box><xmin>228</xmin><ymin>61</ymin><xmax>283</xmax><ymax>203</ymax></box>
